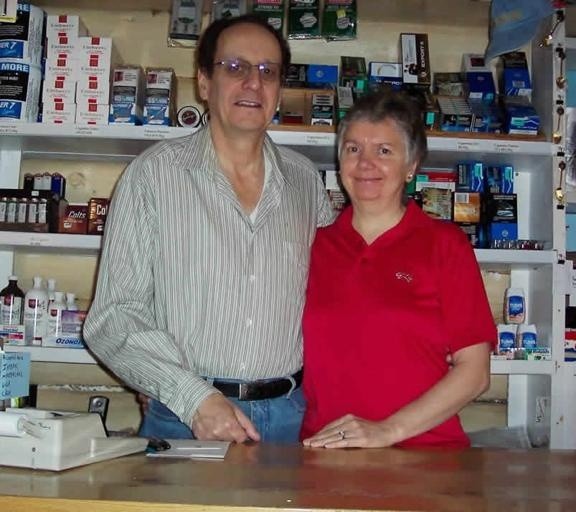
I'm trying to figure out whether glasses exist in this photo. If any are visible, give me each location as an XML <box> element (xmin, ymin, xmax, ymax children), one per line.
<box><xmin>212</xmin><ymin>59</ymin><xmax>281</xmax><ymax>82</ymax></box>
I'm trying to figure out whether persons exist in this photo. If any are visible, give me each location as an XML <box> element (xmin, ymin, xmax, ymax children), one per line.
<box><xmin>137</xmin><ymin>91</ymin><xmax>498</xmax><ymax>449</ymax></box>
<box><xmin>82</xmin><ymin>15</ymin><xmax>455</xmax><ymax>448</ymax></box>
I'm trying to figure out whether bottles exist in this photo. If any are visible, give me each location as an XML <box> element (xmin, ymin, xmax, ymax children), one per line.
<box><xmin>47</xmin><ymin>291</ymin><xmax>78</xmax><ymax>337</ymax></box>
<box><xmin>24</xmin><ymin>276</ymin><xmax>47</xmax><ymax>340</ymax></box>
<box><xmin>0</xmin><ymin>276</ymin><xmax>25</xmax><ymax>324</ymax></box>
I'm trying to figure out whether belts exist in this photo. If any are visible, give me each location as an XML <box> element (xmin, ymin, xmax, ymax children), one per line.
<box><xmin>204</xmin><ymin>367</ymin><xmax>303</xmax><ymax>401</ymax></box>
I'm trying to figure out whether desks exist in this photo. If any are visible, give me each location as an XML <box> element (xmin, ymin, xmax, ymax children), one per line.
<box><xmin>1</xmin><ymin>436</ymin><xmax>576</xmax><ymax>511</ymax></box>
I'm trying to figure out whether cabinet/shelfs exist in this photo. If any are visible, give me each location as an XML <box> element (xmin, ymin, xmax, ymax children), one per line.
<box><xmin>1</xmin><ymin>10</ymin><xmax>575</xmax><ymax>447</ymax></box>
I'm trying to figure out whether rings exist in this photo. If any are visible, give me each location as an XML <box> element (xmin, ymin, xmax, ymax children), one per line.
<box><xmin>339</xmin><ymin>431</ymin><xmax>345</xmax><ymax>440</ymax></box>
